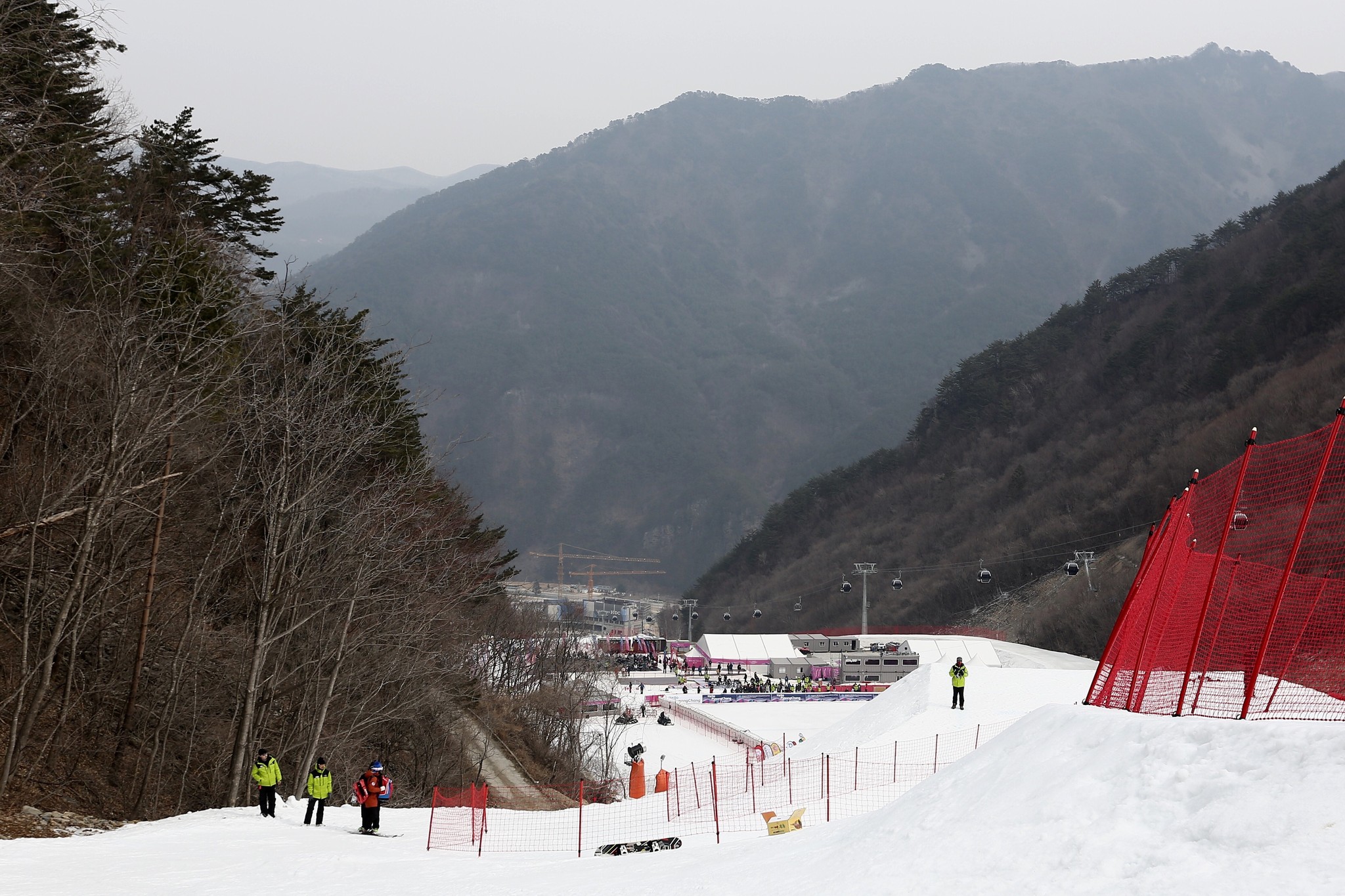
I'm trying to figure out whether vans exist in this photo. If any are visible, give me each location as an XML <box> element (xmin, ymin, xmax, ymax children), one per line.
<box><xmin>870</xmin><ymin>642</ymin><xmax>887</xmax><ymax>652</ymax></box>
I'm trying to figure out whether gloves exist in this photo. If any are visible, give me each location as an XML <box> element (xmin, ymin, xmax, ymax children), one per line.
<box><xmin>954</xmin><ymin>669</ymin><xmax>958</xmax><ymax>675</ymax></box>
<box><xmin>328</xmin><ymin>793</ymin><xmax>331</xmax><ymax>800</ymax></box>
<box><xmin>380</xmin><ymin>786</ymin><xmax>385</xmax><ymax>792</ymax></box>
<box><xmin>309</xmin><ymin>795</ymin><xmax>313</xmax><ymax>801</ymax></box>
<box><xmin>959</xmin><ymin>670</ymin><xmax>964</xmax><ymax>676</ymax></box>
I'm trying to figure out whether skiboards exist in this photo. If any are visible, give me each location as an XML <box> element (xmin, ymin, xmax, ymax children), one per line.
<box><xmin>349</xmin><ymin>831</ymin><xmax>404</xmax><ymax>838</ymax></box>
<box><xmin>298</xmin><ymin>822</ymin><xmax>326</xmax><ymax>827</ymax></box>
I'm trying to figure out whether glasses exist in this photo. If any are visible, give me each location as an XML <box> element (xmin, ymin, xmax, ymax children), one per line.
<box><xmin>375</xmin><ymin>770</ymin><xmax>381</xmax><ymax>772</ymax></box>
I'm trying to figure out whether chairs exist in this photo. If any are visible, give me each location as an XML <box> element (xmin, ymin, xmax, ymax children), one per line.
<box><xmin>536</xmin><ymin>655</ymin><xmax>655</xmax><ymax>673</ymax></box>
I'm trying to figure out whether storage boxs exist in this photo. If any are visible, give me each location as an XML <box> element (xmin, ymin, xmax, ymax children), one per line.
<box><xmin>762</xmin><ymin>807</ymin><xmax>807</xmax><ymax>836</ymax></box>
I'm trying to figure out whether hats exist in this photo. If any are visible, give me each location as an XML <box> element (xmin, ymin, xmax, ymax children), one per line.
<box><xmin>317</xmin><ymin>757</ymin><xmax>326</xmax><ymax>764</ymax></box>
<box><xmin>957</xmin><ymin>657</ymin><xmax>962</xmax><ymax>662</ymax></box>
<box><xmin>258</xmin><ymin>749</ymin><xmax>268</xmax><ymax>755</ymax></box>
<box><xmin>371</xmin><ymin>761</ymin><xmax>383</xmax><ymax>770</ymax></box>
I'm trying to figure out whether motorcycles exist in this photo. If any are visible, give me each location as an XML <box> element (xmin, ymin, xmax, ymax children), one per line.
<box><xmin>657</xmin><ymin>715</ymin><xmax>672</xmax><ymax>726</ymax></box>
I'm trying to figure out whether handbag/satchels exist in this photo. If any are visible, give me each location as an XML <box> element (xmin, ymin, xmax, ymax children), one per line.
<box><xmin>351</xmin><ymin>790</ymin><xmax>361</xmax><ymax>806</ymax></box>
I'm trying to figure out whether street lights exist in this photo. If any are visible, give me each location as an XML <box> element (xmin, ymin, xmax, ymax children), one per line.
<box><xmin>853</xmin><ymin>561</ymin><xmax>877</xmax><ymax>634</ymax></box>
<box><xmin>682</xmin><ymin>598</ymin><xmax>699</xmax><ymax>642</ymax></box>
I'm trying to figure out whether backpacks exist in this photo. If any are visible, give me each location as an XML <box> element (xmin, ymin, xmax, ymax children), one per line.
<box><xmin>378</xmin><ymin>774</ymin><xmax>393</xmax><ymax>802</ymax></box>
<box><xmin>352</xmin><ymin>775</ymin><xmax>378</xmax><ymax>804</ymax></box>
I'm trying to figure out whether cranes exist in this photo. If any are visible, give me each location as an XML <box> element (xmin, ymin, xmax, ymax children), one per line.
<box><xmin>569</xmin><ymin>564</ymin><xmax>667</xmax><ymax>603</ymax></box>
<box><xmin>529</xmin><ymin>543</ymin><xmax>661</xmax><ymax>598</ymax></box>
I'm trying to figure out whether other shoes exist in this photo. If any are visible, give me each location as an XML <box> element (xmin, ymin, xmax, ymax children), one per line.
<box><xmin>373</xmin><ymin>828</ymin><xmax>379</xmax><ymax>832</ymax></box>
<box><xmin>301</xmin><ymin>823</ymin><xmax>310</xmax><ymax>827</ymax></box>
<box><xmin>268</xmin><ymin>810</ymin><xmax>275</xmax><ymax>818</ymax></box>
<box><xmin>358</xmin><ymin>826</ymin><xmax>365</xmax><ymax>832</ymax></box>
<box><xmin>952</xmin><ymin>703</ymin><xmax>956</xmax><ymax>709</ymax></box>
<box><xmin>317</xmin><ymin>824</ymin><xmax>326</xmax><ymax>827</ymax></box>
<box><xmin>262</xmin><ymin>813</ymin><xmax>267</xmax><ymax>818</ymax></box>
<box><xmin>960</xmin><ymin>706</ymin><xmax>964</xmax><ymax>710</ymax></box>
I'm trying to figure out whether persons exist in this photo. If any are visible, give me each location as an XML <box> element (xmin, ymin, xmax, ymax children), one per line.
<box><xmin>615</xmin><ymin>666</ymin><xmax>619</xmax><ymax>675</ymax></box>
<box><xmin>628</xmin><ymin>666</ymin><xmax>630</xmax><ymax>676</ymax></box>
<box><xmin>660</xmin><ymin>711</ymin><xmax>665</xmax><ymax>717</ymax></box>
<box><xmin>818</xmin><ymin>678</ymin><xmax>836</xmax><ymax>691</ymax></box>
<box><xmin>949</xmin><ymin>657</ymin><xmax>968</xmax><ymax>709</ymax></box>
<box><xmin>251</xmin><ymin>749</ymin><xmax>282</xmax><ymax>817</ymax></box>
<box><xmin>628</xmin><ymin>651</ymin><xmax>658</xmax><ymax>671</ymax></box>
<box><xmin>572</xmin><ymin>651</ymin><xmax>587</xmax><ymax>658</ymax></box>
<box><xmin>358</xmin><ymin>760</ymin><xmax>385</xmax><ymax>832</ymax></box>
<box><xmin>844</xmin><ymin>683</ymin><xmax>861</xmax><ymax>692</ymax></box>
<box><xmin>865</xmin><ymin>683</ymin><xmax>868</xmax><ymax>692</ymax></box>
<box><xmin>303</xmin><ymin>757</ymin><xmax>332</xmax><ymax>826</ymax></box>
<box><xmin>597</xmin><ymin>664</ymin><xmax>601</xmax><ymax>674</ymax></box>
<box><xmin>795</xmin><ymin>673</ymin><xmax>812</xmax><ymax>692</ymax></box>
<box><xmin>629</xmin><ymin>682</ymin><xmax>632</xmax><ymax>693</ymax></box>
<box><xmin>640</xmin><ymin>704</ymin><xmax>645</xmax><ymax>717</ymax></box>
<box><xmin>606</xmin><ymin>661</ymin><xmax>609</xmax><ymax>669</ymax></box>
<box><xmin>622</xmin><ymin>667</ymin><xmax>625</xmax><ymax>677</ymax></box>
<box><xmin>659</xmin><ymin>658</ymin><xmax>795</xmax><ymax>693</ymax></box>
<box><xmin>638</xmin><ymin>683</ymin><xmax>645</xmax><ymax>694</ymax></box>
<box><xmin>615</xmin><ymin>648</ymin><xmax>619</xmax><ymax>656</ymax></box>
